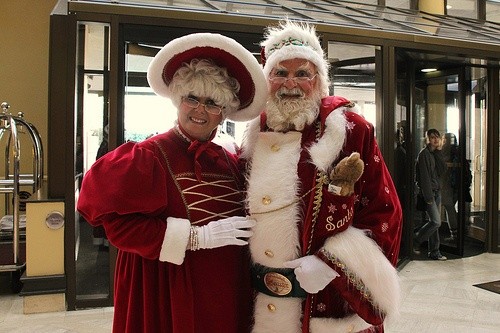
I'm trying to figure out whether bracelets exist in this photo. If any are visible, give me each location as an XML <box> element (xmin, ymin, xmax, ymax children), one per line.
<box><xmin>190</xmin><ymin>226</ymin><xmax>200</xmax><ymax>251</ymax></box>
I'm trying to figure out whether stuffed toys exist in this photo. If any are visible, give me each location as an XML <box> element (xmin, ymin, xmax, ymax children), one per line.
<box><xmin>329</xmin><ymin>151</ymin><xmax>364</xmax><ymax>197</ymax></box>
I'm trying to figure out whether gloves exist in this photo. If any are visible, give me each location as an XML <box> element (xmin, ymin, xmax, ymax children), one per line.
<box><xmin>283</xmin><ymin>254</ymin><xmax>339</xmax><ymax>294</ymax></box>
<box><xmin>196</xmin><ymin>216</ymin><xmax>256</xmax><ymax>250</ymax></box>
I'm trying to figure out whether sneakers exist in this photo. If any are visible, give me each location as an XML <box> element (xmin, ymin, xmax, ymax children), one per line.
<box><xmin>413</xmin><ymin>242</ymin><xmax>421</xmax><ymax>254</ymax></box>
<box><xmin>431</xmin><ymin>250</ymin><xmax>447</xmax><ymax>261</ymax></box>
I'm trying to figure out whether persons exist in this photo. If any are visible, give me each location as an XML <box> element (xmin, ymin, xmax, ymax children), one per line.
<box><xmin>77</xmin><ymin>32</ymin><xmax>269</xmax><ymax>333</ymax></box>
<box><xmin>411</xmin><ymin>128</ymin><xmax>449</xmax><ymax>261</ymax></box>
<box><xmin>238</xmin><ymin>12</ymin><xmax>403</xmax><ymax>333</ymax></box>
<box><xmin>441</xmin><ymin>132</ymin><xmax>473</xmax><ymax>245</ymax></box>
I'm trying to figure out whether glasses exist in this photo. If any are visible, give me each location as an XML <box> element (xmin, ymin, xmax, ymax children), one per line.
<box><xmin>268</xmin><ymin>72</ymin><xmax>317</xmax><ymax>84</ymax></box>
<box><xmin>183</xmin><ymin>96</ymin><xmax>221</xmax><ymax>116</ymax></box>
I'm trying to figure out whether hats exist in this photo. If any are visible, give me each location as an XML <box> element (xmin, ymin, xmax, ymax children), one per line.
<box><xmin>148</xmin><ymin>32</ymin><xmax>268</xmax><ymax>122</ymax></box>
<box><xmin>261</xmin><ymin>19</ymin><xmax>331</xmax><ymax>97</ymax></box>
